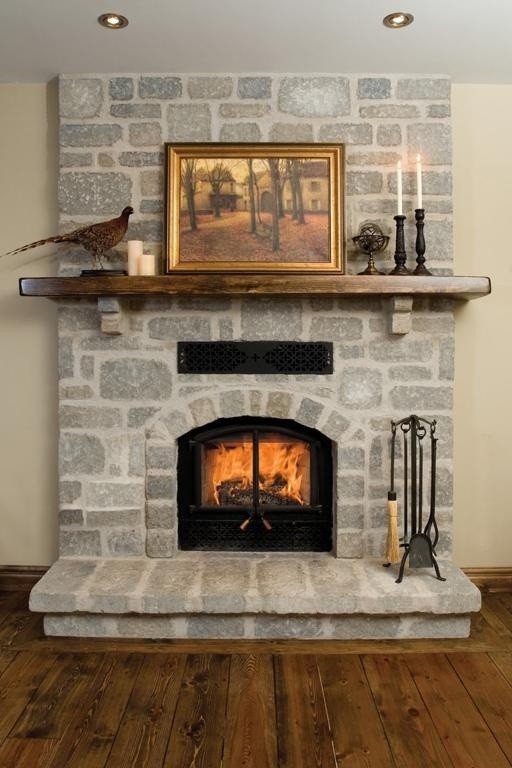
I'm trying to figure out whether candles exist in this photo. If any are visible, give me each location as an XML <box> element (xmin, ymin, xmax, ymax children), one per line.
<box><xmin>396</xmin><ymin>153</ymin><xmax>423</xmax><ymax>217</ymax></box>
<box><xmin>127</xmin><ymin>240</ymin><xmax>154</xmax><ymax>276</ymax></box>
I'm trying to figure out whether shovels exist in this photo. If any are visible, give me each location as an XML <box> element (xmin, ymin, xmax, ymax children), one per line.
<box><xmin>409</xmin><ymin>427</ymin><xmax>433</xmax><ymax>568</ymax></box>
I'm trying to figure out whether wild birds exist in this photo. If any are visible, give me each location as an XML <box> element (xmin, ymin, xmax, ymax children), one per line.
<box><xmin>0</xmin><ymin>206</ymin><xmax>137</xmax><ymax>271</ymax></box>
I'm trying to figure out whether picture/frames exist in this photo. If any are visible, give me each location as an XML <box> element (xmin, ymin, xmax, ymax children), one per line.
<box><xmin>163</xmin><ymin>142</ymin><xmax>346</xmax><ymax>275</ymax></box>
